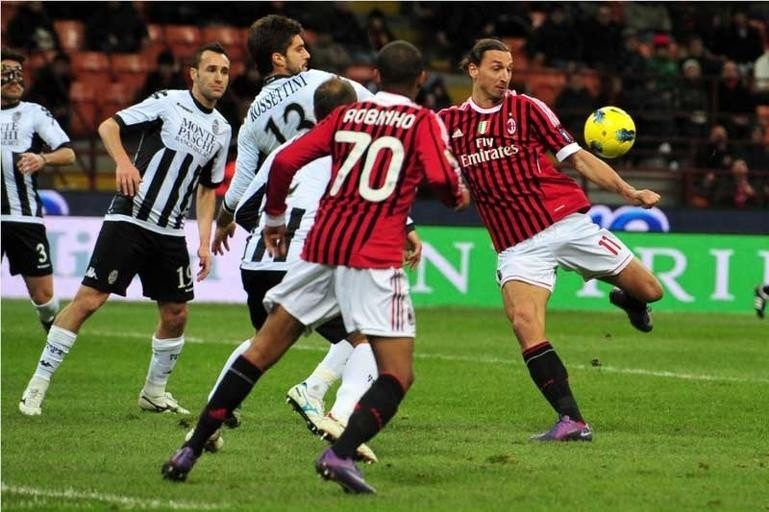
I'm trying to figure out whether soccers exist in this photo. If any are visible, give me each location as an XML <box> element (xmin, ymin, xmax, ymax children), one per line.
<box><xmin>584</xmin><ymin>105</ymin><xmax>637</xmax><ymax>160</ymax></box>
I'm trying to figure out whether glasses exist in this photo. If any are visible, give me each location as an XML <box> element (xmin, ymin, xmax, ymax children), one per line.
<box><xmin>1</xmin><ymin>64</ymin><xmax>24</xmax><ymax>87</ymax></box>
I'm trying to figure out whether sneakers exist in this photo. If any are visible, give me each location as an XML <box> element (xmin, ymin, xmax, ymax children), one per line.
<box><xmin>315</xmin><ymin>447</ymin><xmax>376</xmax><ymax>495</ymax></box>
<box><xmin>222</xmin><ymin>409</ymin><xmax>241</xmax><ymax>428</ymax></box>
<box><xmin>19</xmin><ymin>376</ymin><xmax>49</xmax><ymax>415</ymax></box>
<box><xmin>610</xmin><ymin>286</ymin><xmax>653</xmax><ymax>332</ymax></box>
<box><xmin>138</xmin><ymin>390</ymin><xmax>189</xmax><ymax>414</ymax></box>
<box><xmin>162</xmin><ymin>447</ymin><xmax>196</xmax><ymax>482</ymax></box>
<box><xmin>185</xmin><ymin>427</ymin><xmax>225</xmax><ymax>452</ymax></box>
<box><xmin>317</xmin><ymin>414</ymin><xmax>378</xmax><ymax>464</ymax></box>
<box><xmin>285</xmin><ymin>383</ymin><xmax>325</xmax><ymax>435</ymax></box>
<box><xmin>529</xmin><ymin>416</ymin><xmax>592</xmax><ymax>441</ymax></box>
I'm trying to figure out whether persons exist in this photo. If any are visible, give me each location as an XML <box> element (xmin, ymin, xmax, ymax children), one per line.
<box><xmin>209</xmin><ymin>14</ymin><xmax>379</xmax><ymax>436</ymax></box>
<box><xmin>232</xmin><ymin>72</ymin><xmax>423</xmax><ymax>464</ymax></box>
<box><xmin>17</xmin><ymin>41</ymin><xmax>234</xmax><ymax>416</ymax></box>
<box><xmin>3</xmin><ymin>0</ymin><xmax>769</xmax><ymax>207</ymax></box>
<box><xmin>1</xmin><ymin>51</ymin><xmax>77</xmax><ymax>335</ymax></box>
<box><xmin>436</xmin><ymin>38</ymin><xmax>666</xmax><ymax>443</ymax></box>
<box><xmin>160</xmin><ymin>40</ymin><xmax>471</xmax><ymax>496</ymax></box>
